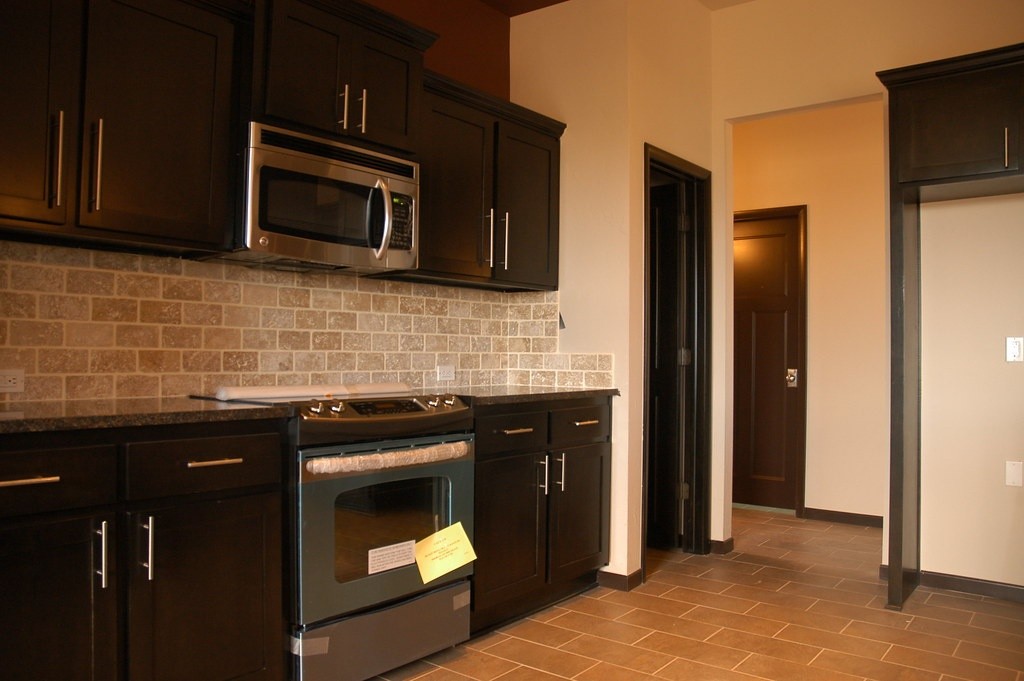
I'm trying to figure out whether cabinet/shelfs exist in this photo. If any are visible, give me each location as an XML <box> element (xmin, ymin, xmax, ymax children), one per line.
<box><xmin>873</xmin><ymin>43</ymin><xmax>1024</xmax><ymax>204</ymax></box>
<box><xmin>0</xmin><ymin>387</ymin><xmax>621</xmax><ymax>681</ymax></box>
<box><xmin>0</xmin><ymin>0</ymin><xmax>565</xmax><ymax>293</ymax></box>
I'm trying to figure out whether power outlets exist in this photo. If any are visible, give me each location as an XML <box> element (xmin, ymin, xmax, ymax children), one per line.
<box><xmin>437</xmin><ymin>366</ymin><xmax>455</xmax><ymax>381</ymax></box>
<box><xmin>1006</xmin><ymin>337</ymin><xmax>1024</xmax><ymax>362</ymax></box>
